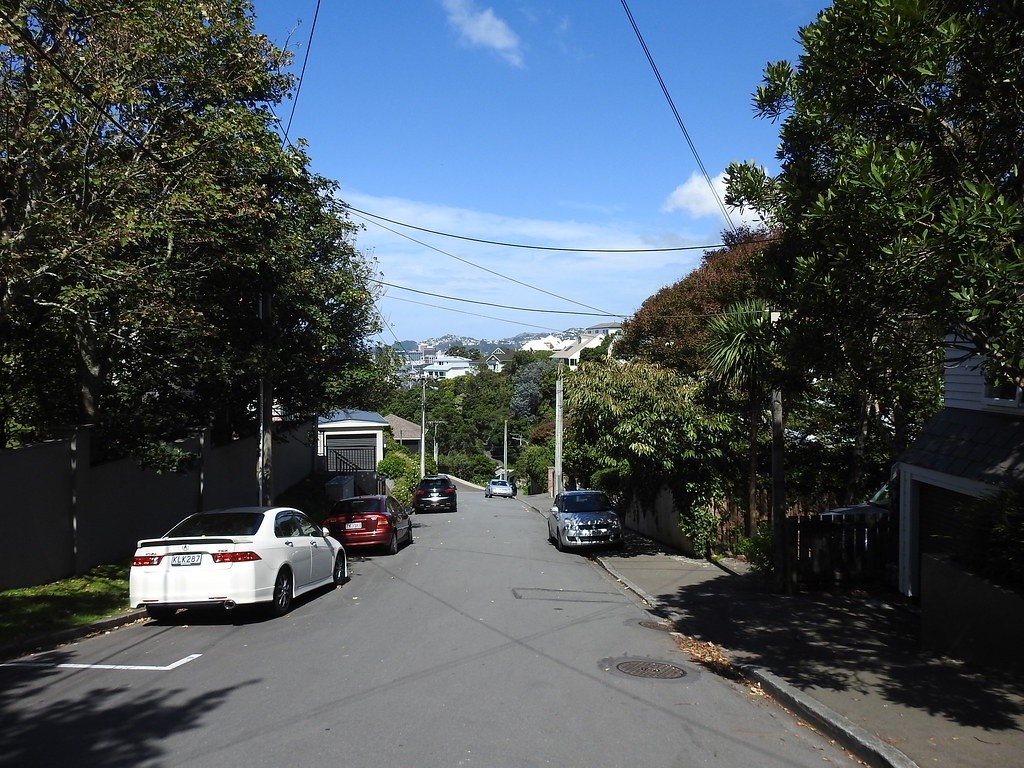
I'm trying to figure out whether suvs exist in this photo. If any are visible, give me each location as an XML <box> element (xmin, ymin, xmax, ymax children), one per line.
<box><xmin>548</xmin><ymin>491</ymin><xmax>624</xmax><ymax>550</ymax></box>
<box><xmin>413</xmin><ymin>476</ymin><xmax>457</xmax><ymax>514</ymax></box>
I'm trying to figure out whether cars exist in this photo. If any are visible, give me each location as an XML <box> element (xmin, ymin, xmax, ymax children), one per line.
<box><xmin>485</xmin><ymin>479</ymin><xmax>515</xmax><ymax>499</ymax></box>
<box><xmin>321</xmin><ymin>494</ymin><xmax>414</xmax><ymax>555</ymax></box>
<box><xmin>129</xmin><ymin>507</ymin><xmax>348</xmax><ymax>619</ymax></box>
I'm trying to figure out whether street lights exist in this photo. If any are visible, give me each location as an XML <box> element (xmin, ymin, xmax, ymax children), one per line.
<box><xmin>411</xmin><ymin>376</ymin><xmax>448</xmax><ymax>479</ymax></box>
